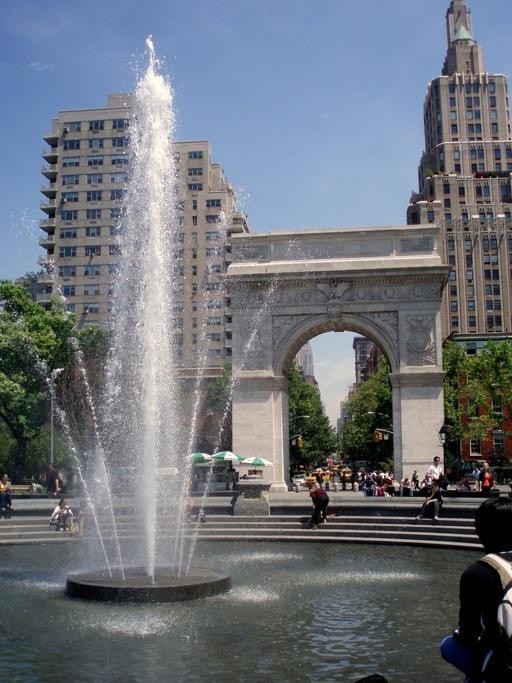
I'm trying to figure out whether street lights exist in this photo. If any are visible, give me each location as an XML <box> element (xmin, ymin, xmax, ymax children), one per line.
<box><xmin>289</xmin><ymin>415</ymin><xmax>310</xmax><ymax>448</ymax></box>
<box><xmin>438</xmin><ymin>426</ymin><xmax>450</xmax><ymax>490</ymax></box>
<box><xmin>367</xmin><ymin>411</ymin><xmax>393</xmax><ymax>442</ymax></box>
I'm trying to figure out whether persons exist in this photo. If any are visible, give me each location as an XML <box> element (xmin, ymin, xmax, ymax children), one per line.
<box><xmin>309</xmin><ymin>484</ymin><xmax>329</xmax><ymax>524</ymax></box>
<box><xmin>417</xmin><ymin>483</ymin><xmax>443</xmax><ymax>520</ymax></box>
<box><xmin>0</xmin><ymin>473</ymin><xmax>13</xmax><ymax>513</ymax></box>
<box><xmin>305</xmin><ymin>458</ymin><xmax>511</xmax><ymax>497</ymax></box>
<box><xmin>30</xmin><ymin>465</ymin><xmax>73</xmax><ymax>532</ymax></box>
<box><xmin>441</xmin><ymin>497</ymin><xmax>512</xmax><ymax>683</ymax></box>
<box><xmin>192</xmin><ymin>468</ymin><xmax>246</xmax><ymax>493</ymax></box>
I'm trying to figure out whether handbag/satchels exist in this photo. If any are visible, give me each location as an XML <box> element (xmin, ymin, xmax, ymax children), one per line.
<box><xmin>439</xmin><ymin>635</ymin><xmax>492</xmax><ymax>679</ymax></box>
<box><xmin>490</xmin><ymin>477</ymin><xmax>494</xmax><ymax>486</ymax></box>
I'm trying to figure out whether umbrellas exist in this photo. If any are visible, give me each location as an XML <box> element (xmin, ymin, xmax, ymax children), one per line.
<box><xmin>212</xmin><ymin>450</ymin><xmax>246</xmax><ymax>463</ymax></box>
<box><xmin>184</xmin><ymin>451</ymin><xmax>213</xmax><ymax>464</ymax></box>
<box><xmin>242</xmin><ymin>456</ymin><xmax>274</xmax><ymax>475</ymax></box>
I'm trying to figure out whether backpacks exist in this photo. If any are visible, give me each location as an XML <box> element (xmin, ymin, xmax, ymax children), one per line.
<box><xmin>480</xmin><ymin>554</ymin><xmax>512</xmax><ymax>654</ymax></box>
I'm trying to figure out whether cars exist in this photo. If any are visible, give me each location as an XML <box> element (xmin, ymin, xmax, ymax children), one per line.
<box><xmin>290</xmin><ymin>464</ymin><xmax>363</xmax><ymax>486</ymax></box>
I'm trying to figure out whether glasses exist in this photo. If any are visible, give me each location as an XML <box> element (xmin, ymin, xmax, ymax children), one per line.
<box><xmin>435</xmin><ymin>460</ymin><xmax>440</xmax><ymax>461</ymax></box>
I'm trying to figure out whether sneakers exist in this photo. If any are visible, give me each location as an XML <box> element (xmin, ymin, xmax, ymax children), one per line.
<box><xmin>417</xmin><ymin>515</ymin><xmax>423</xmax><ymax>519</ymax></box>
<box><xmin>434</xmin><ymin>516</ymin><xmax>439</xmax><ymax>520</ymax></box>
